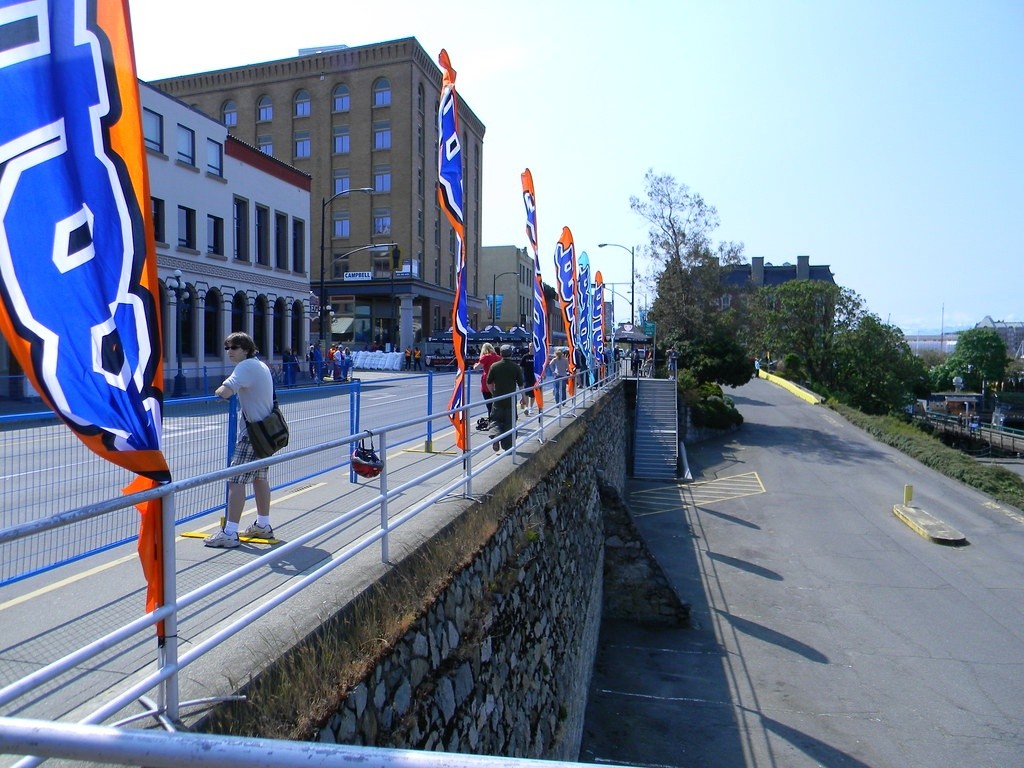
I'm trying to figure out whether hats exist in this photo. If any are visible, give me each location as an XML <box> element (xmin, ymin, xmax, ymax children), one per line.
<box><xmin>556</xmin><ymin>348</ymin><xmax>563</xmax><ymax>355</ymax></box>
<box><xmin>500</xmin><ymin>345</ymin><xmax>512</xmax><ymax>356</ymax></box>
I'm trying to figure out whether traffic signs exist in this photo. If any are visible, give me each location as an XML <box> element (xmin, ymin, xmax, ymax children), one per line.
<box><xmin>644</xmin><ymin>321</ymin><xmax>655</xmax><ymax>337</ymax></box>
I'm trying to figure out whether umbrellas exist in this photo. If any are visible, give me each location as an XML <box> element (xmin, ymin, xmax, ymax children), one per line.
<box><xmin>604</xmin><ymin>322</ymin><xmax>653</xmax><ymax>349</ymax></box>
<box><xmin>427</xmin><ymin>323</ymin><xmax>534</xmax><ymax>355</ymax></box>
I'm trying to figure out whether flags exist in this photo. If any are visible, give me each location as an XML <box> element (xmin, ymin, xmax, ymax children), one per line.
<box><xmin>435</xmin><ymin>49</ymin><xmax>468</xmax><ymax>453</ymax></box>
<box><xmin>0</xmin><ymin>1</ymin><xmax>172</xmax><ymax>648</ymax></box>
<box><xmin>578</xmin><ymin>251</ymin><xmax>596</xmax><ymax>386</ymax></box>
<box><xmin>595</xmin><ymin>271</ymin><xmax>604</xmax><ymax>378</ymax></box>
<box><xmin>520</xmin><ymin>168</ymin><xmax>548</xmax><ymax>412</ymax></box>
<box><xmin>552</xmin><ymin>226</ymin><xmax>578</xmax><ymax>395</ymax></box>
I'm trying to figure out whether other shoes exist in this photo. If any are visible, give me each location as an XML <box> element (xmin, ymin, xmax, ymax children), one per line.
<box><xmin>505</xmin><ymin>448</ymin><xmax>512</xmax><ymax>455</ymax></box>
<box><xmin>493</xmin><ymin>435</ymin><xmax>500</xmax><ymax>452</ymax></box>
<box><xmin>523</xmin><ymin>407</ymin><xmax>530</xmax><ymax>417</ymax></box>
<box><xmin>528</xmin><ymin>408</ymin><xmax>534</xmax><ymax>414</ymax></box>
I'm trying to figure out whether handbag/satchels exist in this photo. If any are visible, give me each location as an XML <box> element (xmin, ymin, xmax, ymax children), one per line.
<box><xmin>247</xmin><ymin>408</ymin><xmax>290</xmax><ymax>458</ymax></box>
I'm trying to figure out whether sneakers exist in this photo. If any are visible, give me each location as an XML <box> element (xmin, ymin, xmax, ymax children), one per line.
<box><xmin>238</xmin><ymin>519</ymin><xmax>275</xmax><ymax>540</ymax></box>
<box><xmin>203</xmin><ymin>526</ymin><xmax>240</xmax><ymax>548</ymax></box>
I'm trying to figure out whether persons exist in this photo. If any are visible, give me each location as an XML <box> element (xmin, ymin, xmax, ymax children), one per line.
<box><xmin>364</xmin><ymin>340</ymin><xmax>400</xmax><ymax>353</ymax></box>
<box><xmin>754</xmin><ymin>357</ymin><xmax>763</xmax><ymax>378</ymax></box>
<box><xmin>519</xmin><ymin>343</ymin><xmax>535</xmax><ymax>416</ymax></box>
<box><xmin>405</xmin><ymin>346</ymin><xmax>422</xmax><ymax>372</ymax></box>
<box><xmin>668</xmin><ymin>347</ymin><xmax>677</xmax><ymax>380</ymax></box>
<box><xmin>473</xmin><ymin>342</ymin><xmax>527</xmax><ymax>455</ymax></box>
<box><xmin>203</xmin><ymin>332</ymin><xmax>274</xmax><ymax>548</ymax></box>
<box><xmin>550</xmin><ymin>345</ymin><xmax>654</xmax><ymax>410</ymax></box>
<box><xmin>283</xmin><ymin>341</ymin><xmax>353</xmax><ymax>385</ymax></box>
<box><xmin>958</xmin><ymin>412</ymin><xmax>983</xmax><ymax>437</ymax></box>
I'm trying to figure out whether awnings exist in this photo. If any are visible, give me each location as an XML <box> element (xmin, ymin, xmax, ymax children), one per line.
<box><xmin>331</xmin><ymin>316</ymin><xmax>355</xmax><ymax>333</ymax></box>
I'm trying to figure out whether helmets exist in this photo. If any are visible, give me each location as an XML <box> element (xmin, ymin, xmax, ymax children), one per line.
<box><xmin>351</xmin><ymin>448</ymin><xmax>384</xmax><ymax>478</ymax></box>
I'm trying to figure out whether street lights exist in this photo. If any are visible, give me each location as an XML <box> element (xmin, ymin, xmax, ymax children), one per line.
<box><xmin>318</xmin><ymin>186</ymin><xmax>375</xmax><ymax>366</ymax></box>
<box><xmin>492</xmin><ymin>271</ymin><xmax>521</xmax><ymax>325</ymax></box>
<box><xmin>597</xmin><ymin>243</ymin><xmax>635</xmax><ymax>370</ymax></box>
<box><xmin>166</xmin><ymin>269</ymin><xmax>191</xmax><ymax>398</ymax></box>
<box><xmin>963</xmin><ymin>401</ymin><xmax>969</xmax><ymax>427</ymax></box>
<box><xmin>627</xmin><ymin>290</ymin><xmax>647</xmax><ymax>323</ymax></box>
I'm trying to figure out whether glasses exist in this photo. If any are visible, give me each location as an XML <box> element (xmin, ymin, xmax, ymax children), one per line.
<box><xmin>223</xmin><ymin>345</ymin><xmax>242</xmax><ymax>351</ymax></box>
